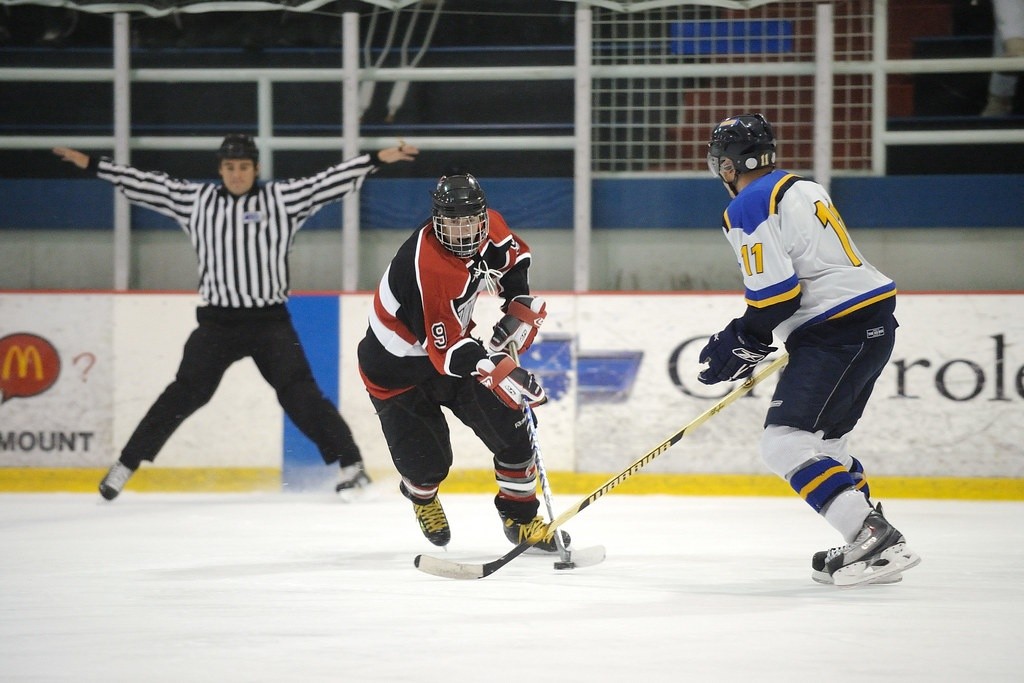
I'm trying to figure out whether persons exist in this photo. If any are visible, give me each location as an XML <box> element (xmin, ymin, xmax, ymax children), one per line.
<box><xmin>984</xmin><ymin>0</ymin><xmax>1024</xmax><ymax>113</ymax></box>
<box><xmin>357</xmin><ymin>172</ymin><xmax>571</xmax><ymax>552</ymax></box>
<box><xmin>697</xmin><ymin>114</ymin><xmax>922</xmax><ymax>587</ymax></box>
<box><xmin>51</xmin><ymin>133</ymin><xmax>419</xmax><ymax>503</ymax></box>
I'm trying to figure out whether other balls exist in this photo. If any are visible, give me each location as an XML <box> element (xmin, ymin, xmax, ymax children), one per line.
<box><xmin>553</xmin><ymin>561</ymin><xmax>578</xmax><ymax>571</ymax></box>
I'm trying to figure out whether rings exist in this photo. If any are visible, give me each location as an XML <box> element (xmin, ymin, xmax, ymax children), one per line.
<box><xmin>398</xmin><ymin>146</ymin><xmax>403</xmax><ymax>152</ymax></box>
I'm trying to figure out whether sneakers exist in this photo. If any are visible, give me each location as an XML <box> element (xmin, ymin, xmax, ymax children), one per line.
<box><xmin>496</xmin><ymin>503</ymin><xmax>571</xmax><ymax>555</ymax></box>
<box><xmin>811</xmin><ymin>502</ymin><xmax>920</xmax><ymax>589</ymax></box>
<box><xmin>97</xmin><ymin>462</ymin><xmax>132</xmax><ymax>506</ymax></box>
<box><xmin>336</xmin><ymin>463</ymin><xmax>374</xmax><ymax>502</ymax></box>
<box><xmin>401</xmin><ymin>487</ymin><xmax>452</xmax><ymax>551</ymax></box>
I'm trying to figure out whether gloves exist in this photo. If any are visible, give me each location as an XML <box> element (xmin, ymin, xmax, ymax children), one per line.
<box><xmin>489</xmin><ymin>295</ymin><xmax>548</xmax><ymax>356</ymax></box>
<box><xmin>471</xmin><ymin>355</ymin><xmax>548</xmax><ymax>410</ymax></box>
<box><xmin>697</xmin><ymin>318</ymin><xmax>779</xmax><ymax>386</ymax></box>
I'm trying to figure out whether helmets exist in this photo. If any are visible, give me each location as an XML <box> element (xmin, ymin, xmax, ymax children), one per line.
<box><xmin>709</xmin><ymin>112</ymin><xmax>776</xmax><ymax>171</ymax></box>
<box><xmin>432</xmin><ymin>175</ymin><xmax>489</xmax><ymax>259</ymax></box>
<box><xmin>216</xmin><ymin>134</ymin><xmax>260</xmax><ymax>161</ymax></box>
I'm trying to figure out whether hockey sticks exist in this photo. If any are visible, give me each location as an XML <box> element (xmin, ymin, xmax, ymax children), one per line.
<box><xmin>508</xmin><ymin>340</ymin><xmax>607</xmax><ymax>570</ymax></box>
<box><xmin>413</xmin><ymin>351</ymin><xmax>790</xmax><ymax>582</ymax></box>
